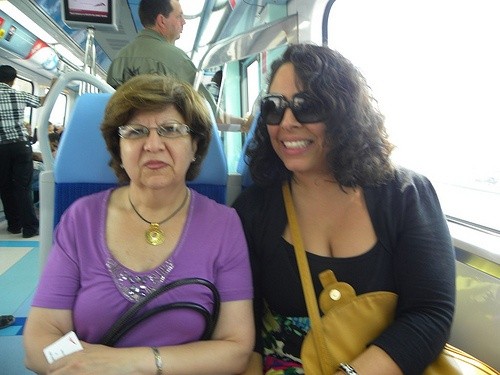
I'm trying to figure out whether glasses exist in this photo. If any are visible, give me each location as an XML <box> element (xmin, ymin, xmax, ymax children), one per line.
<box><xmin>114</xmin><ymin>119</ymin><xmax>196</xmax><ymax>141</ymax></box>
<box><xmin>256</xmin><ymin>90</ymin><xmax>328</xmax><ymax>126</ymax></box>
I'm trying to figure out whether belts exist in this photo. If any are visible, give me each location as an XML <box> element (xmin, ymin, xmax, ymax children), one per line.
<box><xmin>0</xmin><ymin>136</ymin><xmax>28</xmax><ymax>145</ymax></box>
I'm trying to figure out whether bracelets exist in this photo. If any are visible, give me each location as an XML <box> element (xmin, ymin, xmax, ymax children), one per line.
<box><xmin>150</xmin><ymin>346</ymin><xmax>163</xmax><ymax>375</ymax></box>
<box><xmin>336</xmin><ymin>363</ymin><xmax>357</xmax><ymax>375</ymax></box>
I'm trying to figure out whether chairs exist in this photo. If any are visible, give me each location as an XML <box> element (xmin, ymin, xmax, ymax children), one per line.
<box><xmin>52</xmin><ymin>91</ymin><xmax>229</xmax><ymax>251</ymax></box>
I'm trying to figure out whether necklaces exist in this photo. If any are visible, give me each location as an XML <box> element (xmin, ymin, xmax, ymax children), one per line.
<box><xmin>127</xmin><ymin>183</ymin><xmax>188</xmax><ymax>246</ymax></box>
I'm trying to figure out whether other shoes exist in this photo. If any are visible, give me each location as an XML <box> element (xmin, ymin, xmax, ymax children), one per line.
<box><xmin>6</xmin><ymin>225</ymin><xmax>21</xmax><ymax>234</ymax></box>
<box><xmin>22</xmin><ymin>225</ymin><xmax>39</xmax><ymax>238</ymax></box>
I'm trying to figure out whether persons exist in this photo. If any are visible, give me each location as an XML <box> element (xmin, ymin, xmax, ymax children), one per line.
<box><xmin>106</xmin><ymin>0</ymin><xmax>249</xmax><ymax>133</ymax></box>
<box><xmin>0</xmin><ymin>65</ymin><xmax>58</xmax><ymax>237</ymax></box>
<box><xmin>25</xmin><ymin>75</ymin><xmax>256</xmax><ymax>375</ymax></box>
<box><xmin>231</xmin><ymin>44</ymin><xmax>456</xmax><ymax>375</ymax></box>
<box><xmin>23</xmin><ymin>120</ymin><xmax>64</xmax><ymax>156</ymax></box>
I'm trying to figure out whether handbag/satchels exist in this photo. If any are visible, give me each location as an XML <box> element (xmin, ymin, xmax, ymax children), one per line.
<box><xmin>282</xmin><ymin>179</ymin><xmax>500</xmax><ymax>375</ymax></box>
